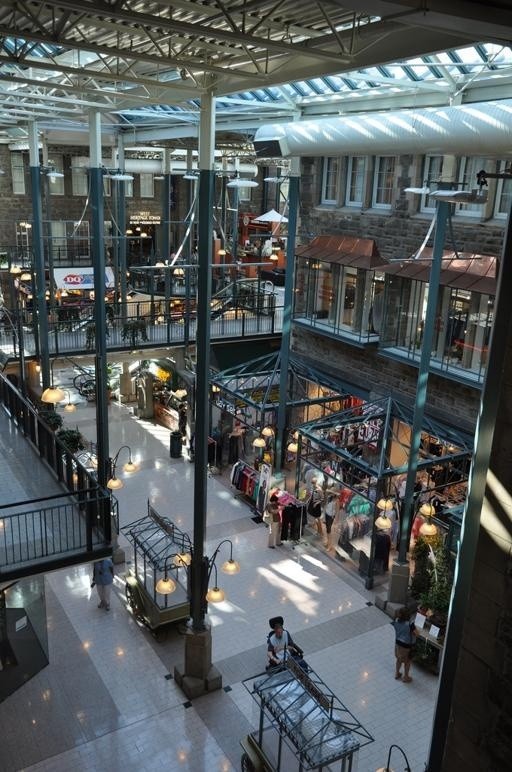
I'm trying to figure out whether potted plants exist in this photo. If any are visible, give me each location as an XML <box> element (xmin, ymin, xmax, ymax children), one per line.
<box><xmin>408</xmin><ymin>528</ymin><xmax>452</xmax><ymax>633</ymax></box>
<box><xmin>105</xmin><ymin>361</ymin><xmax>113</xmax><ymax>405</ymax></box>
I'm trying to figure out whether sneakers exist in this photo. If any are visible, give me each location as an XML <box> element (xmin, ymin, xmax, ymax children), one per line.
<box><xmin>98</xmin><ymin>602</ymin><xmax>110</xmax><ymax>612</ymax></box>
<box><xmin>394</xmin><ymin>672</ymin><xmax>412</xmax><ymax>683</ymax></box>
<box><xmin>269</xmin><ymin>542</ymin><xmax>284</xmax><ymax>548</ymax></box>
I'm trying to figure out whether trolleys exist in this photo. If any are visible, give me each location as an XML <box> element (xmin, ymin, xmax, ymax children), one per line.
<box><xmin>117</xmin><ymin>496</ymin><xmax>194</xmax><ymax>644</ymax></box>
<box><xmin>236</xmin><ymin>652</ymin><xmax>378</xmax><ymax>772</ymax></box>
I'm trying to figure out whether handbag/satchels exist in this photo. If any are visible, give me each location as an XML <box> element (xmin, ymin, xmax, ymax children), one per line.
<box><xmin>408</xmin><ymin>649</ymin><xmax>416</xmax><ymax>659</ymax></box>
<box><xmin>262</xmin><ymin>510</ymin><xmax>273</xmax><ymax>525</ymax></box>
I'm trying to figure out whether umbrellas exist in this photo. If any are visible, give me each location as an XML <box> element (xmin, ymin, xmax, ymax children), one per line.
<box><xmin>251</xmin><ymin>208</ymin><xmax>289</xmax><ymax>239</ymax></box>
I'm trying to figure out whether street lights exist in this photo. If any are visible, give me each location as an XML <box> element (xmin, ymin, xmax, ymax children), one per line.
<box><xmin>153</xmin><ymin>530</ymin><xmax>242</xmax><ymax>702</ymax></box>
<box><xmin>251</xmin><ymin>423</ymin><xmax>305</xmax><ymax>477</ymax></box>
<box><xmin>109</xmin><ymin>445</ymin><xmax>141</xmax><ymax>493</ymax></box>
<box><xmin>371</xmin><ymin>741</ymin><xmax>412</xmax><ymax>772</ymax></box>
<box><xmin>40</xmin><ymin>383</ymin><xmax>75</xmax><ymax>417</ymax></box>
<box><xmin>372</xmin><ymin>479</ymin><xmax>441</xmax><ymax>603</ymax></box>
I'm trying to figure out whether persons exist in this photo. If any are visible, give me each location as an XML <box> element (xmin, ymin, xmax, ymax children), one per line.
<box><xmin>304</xmin><ymin>477</ymin><xmax>326</xmax><ymax>538</ymax></box>
<box><xmin>90</xmin><ymin>558</ymin><xmax>115</xmax><ymax>612</ymax></box>
<box><xmin>319</xmin><ymin>492</ymin><xmax>340</xmax><ymax>551</ymax></box>
<box><xmin>178</xmin><ymin>403</ymin><xmax>186</xmax><ymax>443</ymax></box>
<box><xmin>394</xmin><ymin>607</ymin><xmax>419</xmax><ymax>683</ymax></box>
<box><xmin>57</xmin><ymin>300</ymin><xmax>73</xmax><ymax>333</ymax></box>
<box><xmin>252</xmin><ymin>241</ymin><xmax>261</xmax><ymax>255</ymax></box>
<box><xmin>266</xmin><ymin>495</ymin><xmax>293</xmax><ymax>549</ymax></box>
<box><xmin>267</xmin><ymin>624</ymin><xmax>309</xmax><ymax>677</ymax></box>
<box><xmin>258</xmin><ymin>479</ymin><xmax>267</xmax><ymax>514</ymax></box>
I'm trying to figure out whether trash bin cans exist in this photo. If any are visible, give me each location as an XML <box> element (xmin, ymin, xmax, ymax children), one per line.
<box><xmin>388</xmin><ymin>564</ymin><xmax>410</xmax><ymax>604</ymax></box>
<box><xmin>170</xmin><ymin>432</ymin><xmax>182</xmax><ymax>458</ymax></box>
